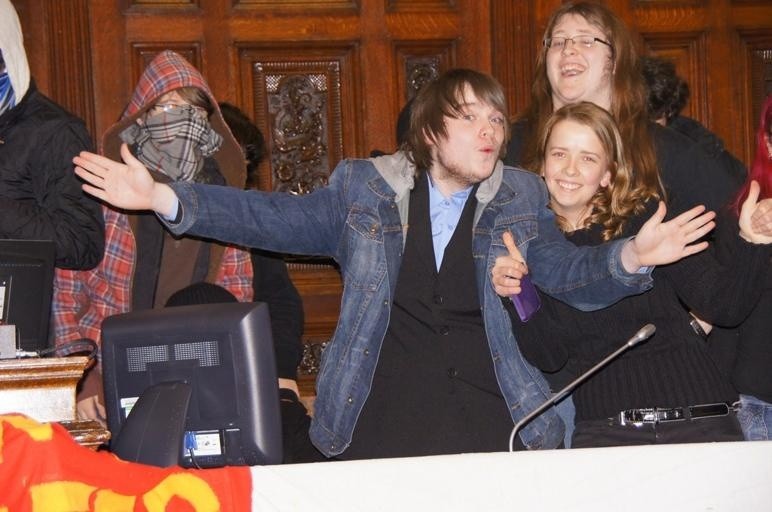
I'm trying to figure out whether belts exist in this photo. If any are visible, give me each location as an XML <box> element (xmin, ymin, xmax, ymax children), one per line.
<box><xmin>621</xmin><ymin>404</ymin><xmax>726</xmax><ymax>423</ymax></box>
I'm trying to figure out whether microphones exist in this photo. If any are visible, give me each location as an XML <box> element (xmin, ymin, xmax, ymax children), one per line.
<box><xmin>505</xmin><ymin>322</ymin><xmax>656</xmax><ymax>452</ymax></box>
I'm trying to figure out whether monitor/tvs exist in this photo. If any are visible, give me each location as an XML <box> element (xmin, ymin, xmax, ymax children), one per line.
<box><xmin>0</xmin><ymin>239</ymin><xmax>56</xmax><ymax>352</ymax></box>
<box><xmin>99</xmin><ymin>301</ymin><xmax>283</xmax><ymax>467</ymax></box>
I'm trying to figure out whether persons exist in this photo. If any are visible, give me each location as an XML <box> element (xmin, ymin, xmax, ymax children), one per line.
<box><xmin>44</xmin><ymin>49</ymin><xmax>253</xmax><ymax>452</ymax></box>
<box><xmin>217</xmin><ymin>102</ymin><xmax>307</xmax><ymax>414</ymax></box>
<box><xmin>163</xmin><ymin>282</ymin><xmax>312</xmax><ymax>464</ymax></box>
<box><xmin>0</xmin><ymin>1</ymin><xmax>105</xmax><ymax>271</ymax></box>
<box><xmin>73</xmin><ymin>68</ymin><xmax>716</xmax><ymax>462</ymax></box>
<box><xmin>491</xmin><ymin>101</ymin><xmax>772</xmax><ymax>449</ymax></box>
<box><xmin>371</xmin><ymin>96</ymin><xmax>417</xmax><ymax>158</ymax></box>
<box><xmin>500</xmin><ymin>2</ymin><xmax>771</xmax><ymax>442</ymax></box>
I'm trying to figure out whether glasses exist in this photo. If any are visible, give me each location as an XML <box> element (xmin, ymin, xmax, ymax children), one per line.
<box><xmin>157</xmin><ymin>102</ymin><xmax>207</xmax><ymax>119</ymax></box>
<box><xmin>547</xmin><ymin>35</ymin><xmax>610</xmax><ymax>49</ymax></box>
